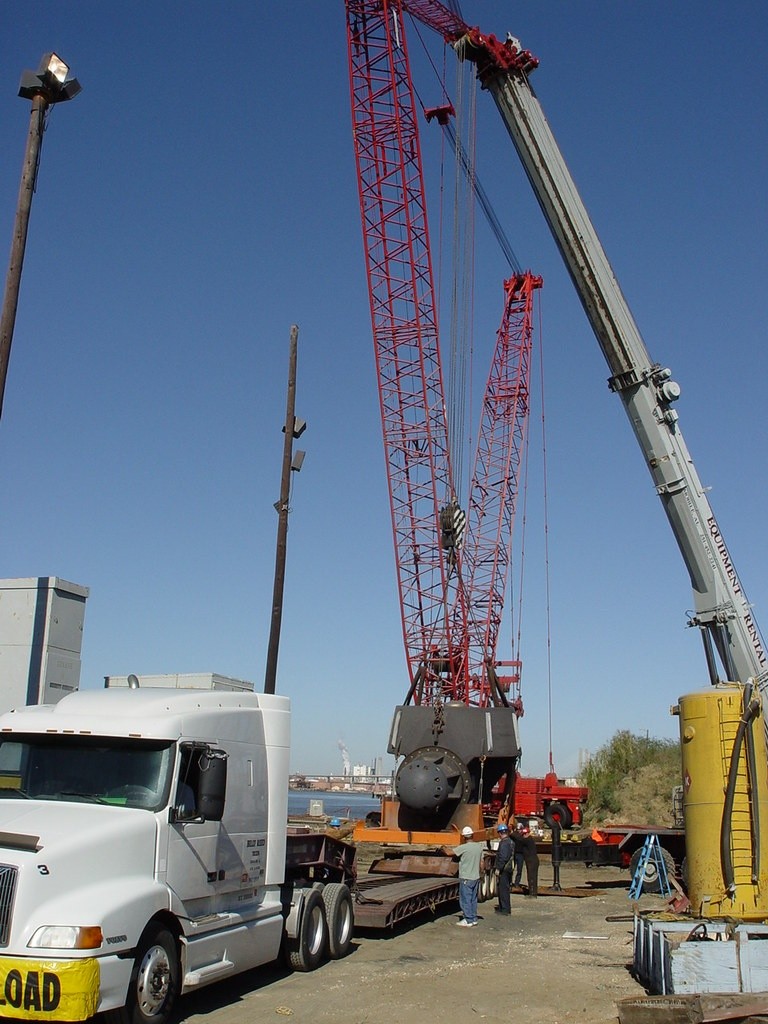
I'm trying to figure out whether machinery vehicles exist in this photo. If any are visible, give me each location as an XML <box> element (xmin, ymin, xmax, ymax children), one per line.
<box><xmin>481</xmin><ymin>766</ymin><xmax>590</xmax><ymax>829</ymax></box>
<box><xmin>454</xmin><ymin>23</ymin><xmax>768</xmax><ymax>924</ymax></box>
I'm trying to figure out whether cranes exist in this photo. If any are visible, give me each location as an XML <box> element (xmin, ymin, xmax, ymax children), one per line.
<box><xmin>334</xmin><ymin>0</ymin><xmax>548</xmax><ymax>846</ymax></box>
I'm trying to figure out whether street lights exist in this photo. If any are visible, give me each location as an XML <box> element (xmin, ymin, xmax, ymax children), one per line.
<box><xmin>0</xmin><ymin>52</ymin><xmax>83</xmax><ymax>416</ymax></box>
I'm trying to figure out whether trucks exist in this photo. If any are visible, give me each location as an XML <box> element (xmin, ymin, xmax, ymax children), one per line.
<box><xmin>598</xmin><ymin>784</ymin><xmax>687</xmax><ymax>894</ymax></box>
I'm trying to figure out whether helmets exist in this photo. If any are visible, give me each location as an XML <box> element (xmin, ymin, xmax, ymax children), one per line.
<box><xmin>521</xmin><ymin>828</ymin><xmax>529</xmax><ymax>834</ymax></box>
<box><xmin>517</xmin><ymin>823</ymin><xmax>523</xmax><ymax>830</ymax></box>
<box><xmin>496</xmin><ymin>824</ymin><xmax>508</xmax><ymax>832</ymax></box>
<box><xmin>329</xmin><ymin>818</ymin><xmax>341</xmax><ymax>826</ymax></box>
<box><xmin>462</xmin><ymin>827</ymin><xmax>474</xmax><ymax>836</ymax></box>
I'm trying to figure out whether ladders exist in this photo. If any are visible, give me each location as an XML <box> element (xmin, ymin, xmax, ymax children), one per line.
<box><xmin>627</xmin><ymin>833</ymin><xmax>673</xmax><ymax>902</ymax></box>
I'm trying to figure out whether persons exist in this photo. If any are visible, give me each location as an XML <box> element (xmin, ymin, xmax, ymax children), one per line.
<box><xmin>329</xmin><ymin>819</ymin><xmax>340</xmax><ymax>829</ymax></box>
<box><xmin>510</xmin><ymin>823</ymin><xmax>540</xmax><ymax>899</ymax></box>
<box><xmin>441</xmin><ymin>827</ymin><xmax>484</xmax><ymax>927</ymax></box>
<box><xmin>493</xmin><ymin>825</ymin><xmax>515</xmax><ymax>916</ymax></box>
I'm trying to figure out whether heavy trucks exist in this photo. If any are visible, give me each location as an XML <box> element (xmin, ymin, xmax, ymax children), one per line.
<box><xmin>0</xmin><ymin>675</ymin><xmax>357</xmax><ymax>1024</ymax></box>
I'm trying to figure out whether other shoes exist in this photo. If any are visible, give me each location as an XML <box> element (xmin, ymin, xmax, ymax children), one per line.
<box><xmin>473</xmin><ymin>922</ymin><xmax>477</xmax><ymax>925</ymax></box>
<box><xmin>497</xmin><ymin>910</ymin><xmax>510</xmax><ymax>916</ymax></box>
<box><xmin>457</xmin><ymin>919</ymin><xmax>473</xmax><ymax>926</ymax></box>
